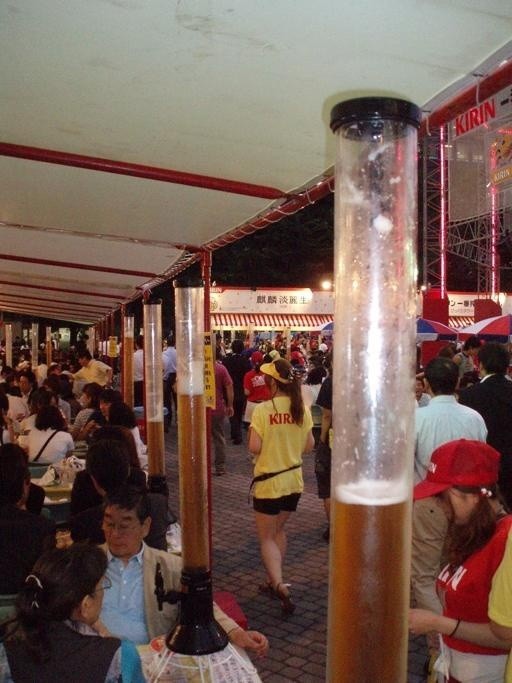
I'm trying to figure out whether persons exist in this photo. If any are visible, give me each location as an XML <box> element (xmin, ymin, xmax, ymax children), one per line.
<box><xmin>70</xmin><ymin>439</ymin><xmax>172</xmax><ymax>576</ymax></box>
<box><xmin>0</xmin><ymin>540</ymin><xmax>144</xmax><ymax>682</ymax></box>
<box><xmin>108</xmin><ymin>403</ymin><xmax>153</xmax><ymax>472</ymax></box>
<box><xmin>0</xmin><ymin>443</ymin><xmax>59</xmax><ymax>590</ymax></box>
<box><xmin>165</xmin><ymin>334</ymin><xmax>179</xmax><ymax>428</ymax></box>
<box><xmin>247</xmin><ymin>360</ymin><xmax>315</xmax><ymax>615</ymax></box>
<box><xmin>75</xmin><ymin>383</ymin><xmax>109</xmax><ymax>434</ymax></box>
<box><xmin>460</xmin><ymin>342</ymin><xmax>510</xmax><ymax>513</ymax></box>
<box><xmin>207</xmin><ymin>352</ymin><xmax>234</xmax><ymax>475</ymax></box>
<box><xmin>410</xmin><ymin>360</ymin><xmax>487</xmax><ymax>673</ymax></box>
<box><xmin>75</xmin><ymin>349</ymin><xmax>116</xmax><ymax>403</ymax></box>
<box><xmin>249</xmin><ymin>328</ymin><xmax>338</xmax><ymax>369</ymax></box>
<box><xmin>20</xmin><ymin>405</ymin><xmax>76</xmax><ymax>467</ymax></box>
<box><xmin>407</xmin><ymin>438</ymin><xmax>512</xmax><ymax>683</ymax></box>
<box><xmin>226</xmin><ymin>338</ymin><xmax>250</xmax><ymax>446</ymax></box>
<box><xmin>133</xmin><ymin>334</ymin><xmax>146</xmax><ymax>403</ymax></box>
<box><xmin>83</xmin><ymin>486</ymin><xmax>269</xmax><ymax>682</ymax></box>
<box><xmin>312</xmin><ymin>349</ymin><xmax>333</xmax><ymax>540</ymax></box>
<box><xmin>0</xmin><ymin>325</ymin><xmax>84</xmax><ymax>432</ymax></box>
<box><xmin>85</xmin><ymin>390</ymin><xmax>123</xmax><ymax>440</ymax></box>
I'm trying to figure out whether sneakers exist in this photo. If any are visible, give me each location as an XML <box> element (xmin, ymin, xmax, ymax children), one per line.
<box><xmin>215</xmin><ymin>465</ymin><xmax>224</xmax><ymax>475</ymax></box>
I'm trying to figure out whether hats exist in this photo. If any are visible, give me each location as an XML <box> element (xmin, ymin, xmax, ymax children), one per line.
<box><xmin>249</xmin><ymin>351</ymin><xmax>263</xmax><ymax>366</ymax></box>
<box><xmin>315</xmin><ymin>344</ymin><xmax>328</xmax><ymax>352</ymax></box>
<box><xmin>260</xmin><ymin>359</ymin><xmax>294</xmax><ymax>384</ymax></box>
<box><xmin>414</xmin><ymin>439</ymin><xmax>500</xmax><ymax>500</ymax></box>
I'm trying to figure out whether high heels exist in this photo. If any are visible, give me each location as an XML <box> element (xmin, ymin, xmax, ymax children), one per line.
<box><xmin>276</xmin><ymin>582</ymin><xmax>297</xmax><ymax>613</ymax></box>
<box><xmin>258</xmin><ymin>580</ymin><xmax>275</xmax><ymax>594</ymax></box>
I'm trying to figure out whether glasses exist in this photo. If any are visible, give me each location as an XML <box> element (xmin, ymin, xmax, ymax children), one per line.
<box><xmin>102</xmin><ymin>519</ymin><xmax>145</xmax><ymax>531</ymax></box>
<box><xmin>80</xmin><ymin>576</ymin><xmax>113</xmax><ymax>603</ymax></box>
